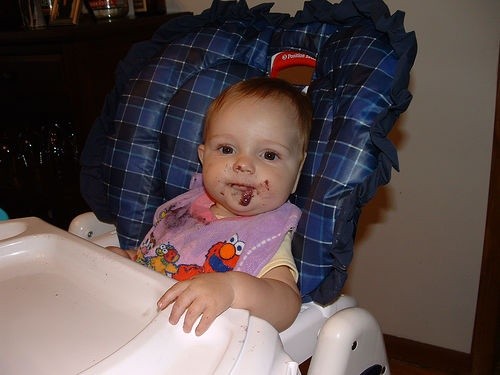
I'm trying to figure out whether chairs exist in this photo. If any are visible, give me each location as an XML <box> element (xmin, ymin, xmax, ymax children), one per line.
<box><xmin>0</xmin><ymin>0</ymin><xmax>417</xmax><ymax>374</ymax></box>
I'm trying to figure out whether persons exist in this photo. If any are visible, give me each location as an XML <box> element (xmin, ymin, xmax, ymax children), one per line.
<box><xmin>103</xmin><ymin>73</ymin><xmax>313</xmax><ymax>337</ymax></box>
